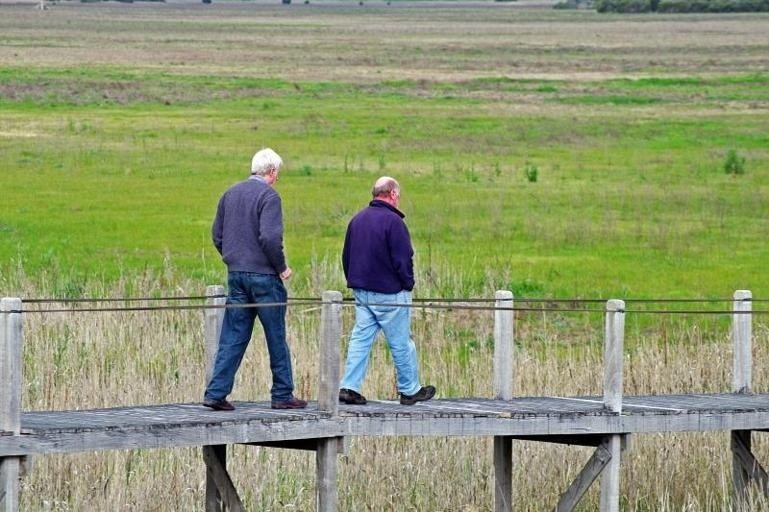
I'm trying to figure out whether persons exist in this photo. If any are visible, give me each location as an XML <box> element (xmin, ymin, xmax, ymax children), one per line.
<box><xmin>201</xmin><ymin>149</ymin><xmax>307</xmax><ymax>411</ymax></box>
<box><xmin>340</xmin><ymin>176</ymin><xmax>436</xmax><ymax>406</ymax></box>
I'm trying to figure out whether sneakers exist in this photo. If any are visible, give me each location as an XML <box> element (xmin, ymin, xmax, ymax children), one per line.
<box><xmin>339</xmin><ymin>389</ymin><xmax>366</xmax><ymax>404</ymax></box>
<box><xmin>271</xmin><ymin>398</ymin><xmax>305</xmax><ymax>409</ymax></box>
<box><xmin>400</xmin><ymin>386</ymin><xmax>435</xmax><ymax>405</ymax></box>
<box><xmin>203</xmin><ymin>398</ymin><xmax>235</xmax><ymax>410</ymax></box>
